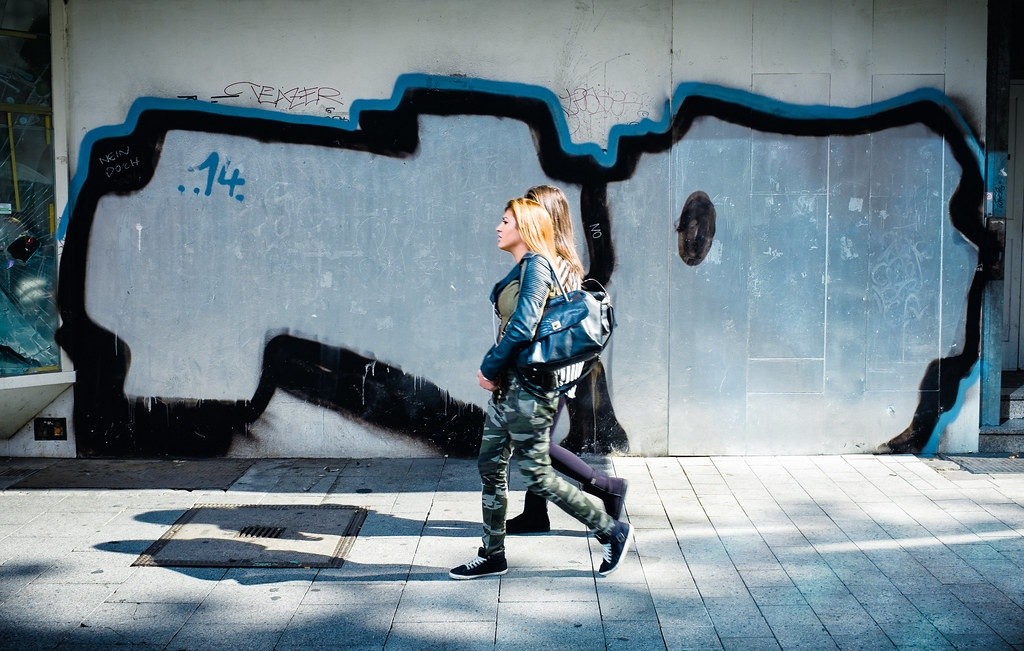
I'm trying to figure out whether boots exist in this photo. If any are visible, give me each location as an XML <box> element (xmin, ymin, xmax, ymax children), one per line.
<box><xmin>582</xmin><ymin>466</ymin><xmax>628</xmax><ymax>520</ymax></box>
<box><xmin>506</xmin><ymin>491</ymin><xmax>550</xmax><ymax>534</ymax></box>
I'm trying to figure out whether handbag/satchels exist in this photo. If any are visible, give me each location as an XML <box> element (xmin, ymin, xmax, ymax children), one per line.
<box><xmin>502</xmin><ymin>252</ymin><xmax>616</xmax><ymax>401</ymax></box>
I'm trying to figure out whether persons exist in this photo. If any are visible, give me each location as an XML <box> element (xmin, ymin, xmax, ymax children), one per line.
<box><xmin>504</xmin><ymin>184</ymin><xmax>628</xmax><ymax>535</ymax></box>
<box><xmin>445</xmin><ymin>197</ymin><xmax>632</xmax><ymax>580</ymax></box>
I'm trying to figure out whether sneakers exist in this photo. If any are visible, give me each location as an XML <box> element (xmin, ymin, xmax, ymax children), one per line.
<box><xmin>592</xmin><ymin>519</ymin><xmax>634</xmax><ymax>576</ymax></box>
<box><xmin>448</xmin><ymin>546</ymin><xmax>509</xmax><ymax>580</ymax></box>
